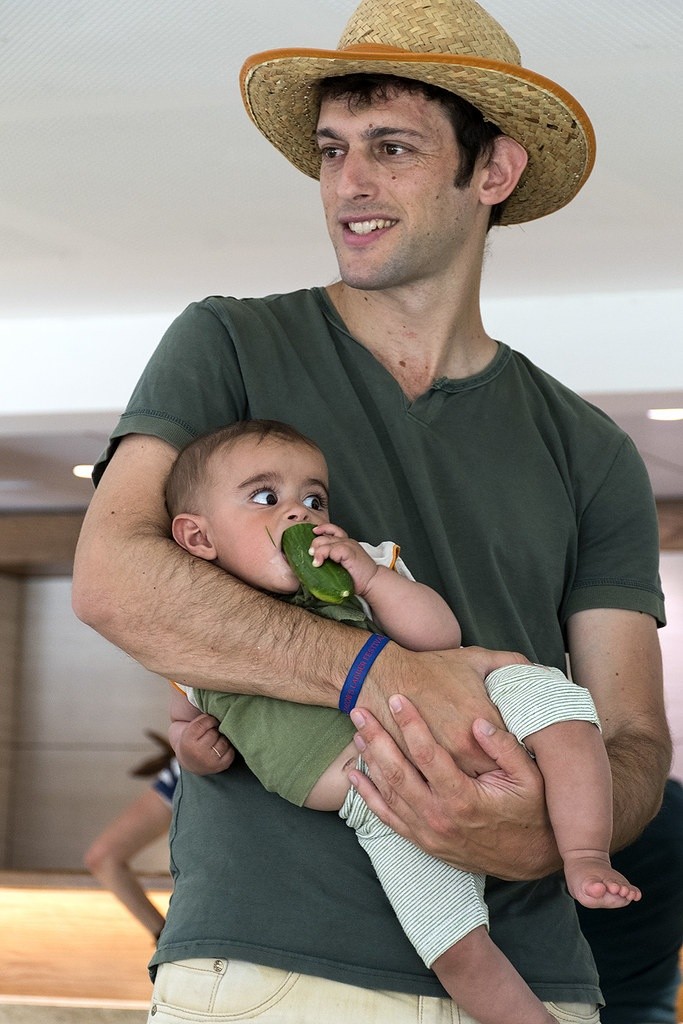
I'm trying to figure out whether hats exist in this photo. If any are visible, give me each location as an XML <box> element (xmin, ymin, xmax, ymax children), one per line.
<box><xmin>236</xmin><ymin>1</ymin><xmax>598</xmax><ymax>227</ymax></box>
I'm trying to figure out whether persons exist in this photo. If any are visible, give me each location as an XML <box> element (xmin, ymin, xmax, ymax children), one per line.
<box><xmin>71</xmin><ymin>0</ymin><xmax>672</xmax><ymax>1024</ymax></box>
<box><xmin>85</xmin><ymin>546</ymin><xmax>683</xmax><ymax>1024</ymax></box>
<box><xmin>161</xmin><ymin>418</ymin><xmax>646</xmax><ymax>1024</ymax></box>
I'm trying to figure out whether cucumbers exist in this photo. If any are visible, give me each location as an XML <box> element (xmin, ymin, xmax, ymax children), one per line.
<box><xmin>282</xmin><ymin>522</ymin><xmax>354</xmax><ymax>604</ymax></box>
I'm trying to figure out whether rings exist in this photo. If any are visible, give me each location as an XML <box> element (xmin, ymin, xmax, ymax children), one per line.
<box><xmin>212</xmin><ymin>746</ymin><xmax>222</xmax><ymax>758</ymax></box>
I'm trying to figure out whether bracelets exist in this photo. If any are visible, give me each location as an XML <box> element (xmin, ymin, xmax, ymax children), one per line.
<box><xmin>338</xmin><ymin>632</ymin><xmax>390</xmax><ymax>714</ymax></box>
